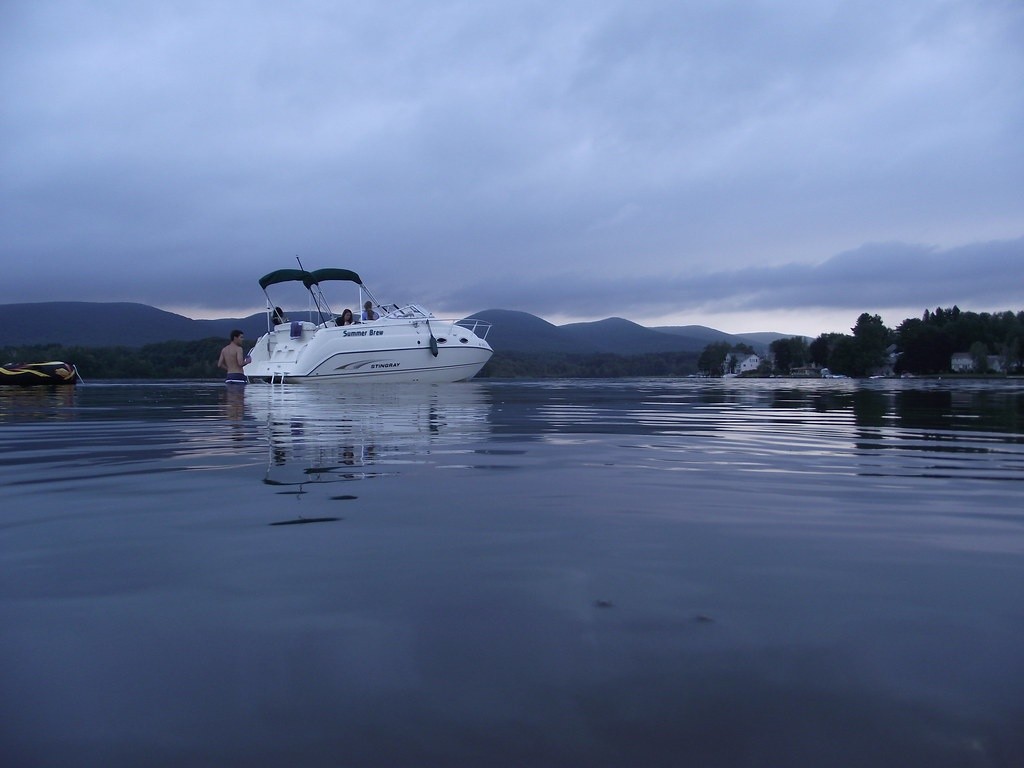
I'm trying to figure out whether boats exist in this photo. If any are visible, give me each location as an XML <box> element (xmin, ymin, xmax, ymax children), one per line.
<box><xmin>241</xmin><ymin>254</ymin><xmax>495</xmax><ymax>384</ymax></box>
<box><xmin>0</xmin><ymin>360</ymin><xmax>78</xmax><ymax>385</ymax></box>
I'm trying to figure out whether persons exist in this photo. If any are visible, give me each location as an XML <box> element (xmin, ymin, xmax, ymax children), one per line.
<box><xmin>218</xmin><ymin>330</ymin><xmax>251</xmax><ymax>383</ymax></box>
<box><xmin>336</xmin><ymin>309</ymin><xmax>353</xmax><ymax>326</ymax></box>
<box><xmin>362</xmin><ymin>301</ymin><xmax>379</xmax><ymax>320</ymax></box>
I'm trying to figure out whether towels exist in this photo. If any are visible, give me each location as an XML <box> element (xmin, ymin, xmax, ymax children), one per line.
<box><xmin>289</xmin><ymin>318</ymin><xmax>303</xmax><ymax>340</ymax></box>
